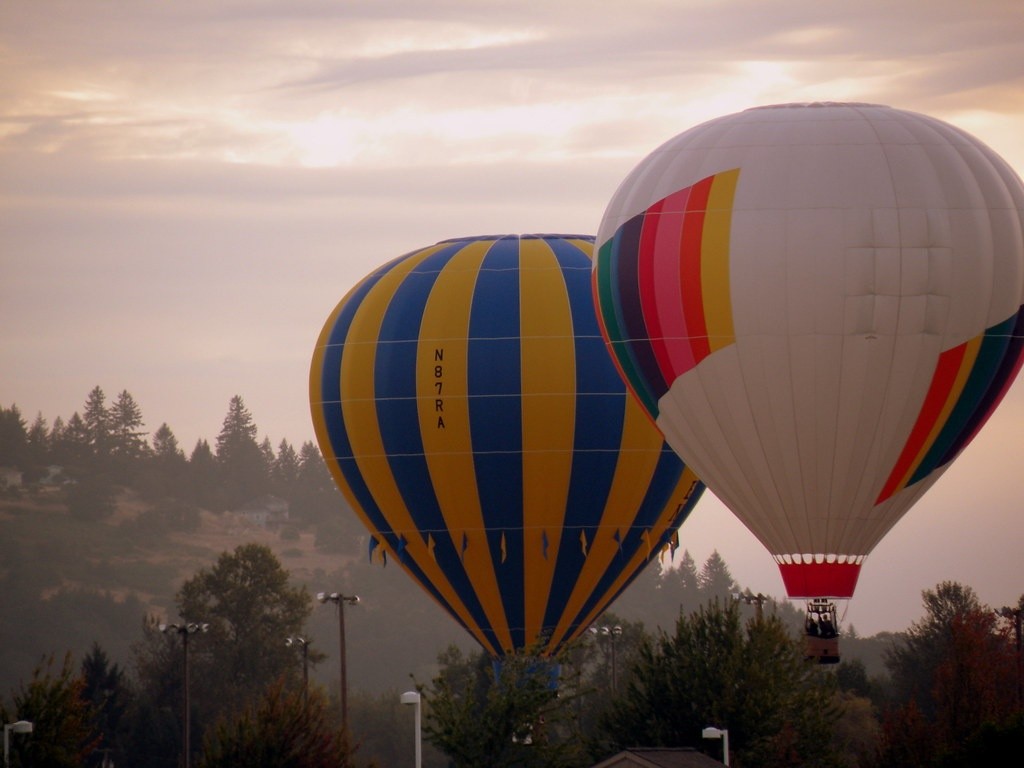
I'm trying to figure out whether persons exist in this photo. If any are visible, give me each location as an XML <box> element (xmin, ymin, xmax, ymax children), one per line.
<box><xmin>818</xmin><ymin>615</ymin><xmax>835</xmax><ymax>638</ymax></box>
<box><xmin>537</xmin><ymin>711</ymin><xmax>544</xmax><ymax>724</ymax></box>
<box><xmin>807</xmin><ymin>619</ymin><xmax>818</xmax><ymax>636</ymax></box>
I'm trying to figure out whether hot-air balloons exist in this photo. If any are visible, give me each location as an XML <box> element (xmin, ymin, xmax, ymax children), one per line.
<box><xmin>308</xmin><ymin>233</ymin><xmax>711</xmax><ymax>745</ymax></box>
<box><xmin>590</xmin><ymin>101</ymin><xmax>1024</xmax><ymax>663</ymax></box>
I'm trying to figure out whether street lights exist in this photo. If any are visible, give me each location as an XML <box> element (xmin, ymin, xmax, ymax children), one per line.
<box><xmin>589</xmin><ymin>626</ymin><xmax>622</xmax><ymax>703</ymax></box>
<box><xmin>316</xmin><ymin>591</ymin><xmax>360</xmax><ymax>768</ymax></box>
<box><xmin>401</xmin><ymin>691</ymin><xmax>422</xmax><ymax>768</ymax></box>
<box><xmin>157</xmin><ymin>621</ymin><xmax>213</xmax><ymax>768</ymax></box>
<box><xmin>702</xmin><ymin>727</ymin><xmax>729</xmax><ymax>768</ymax></box>
<box><xmin>3</xmin><ymin>720</ymin><xmax>33</xmax><ymax>768</ymax></box>
<box><xmin>284</xmin><ymin>634</ymin><xmax>316</xmax><ymax>768</ymax></box>
